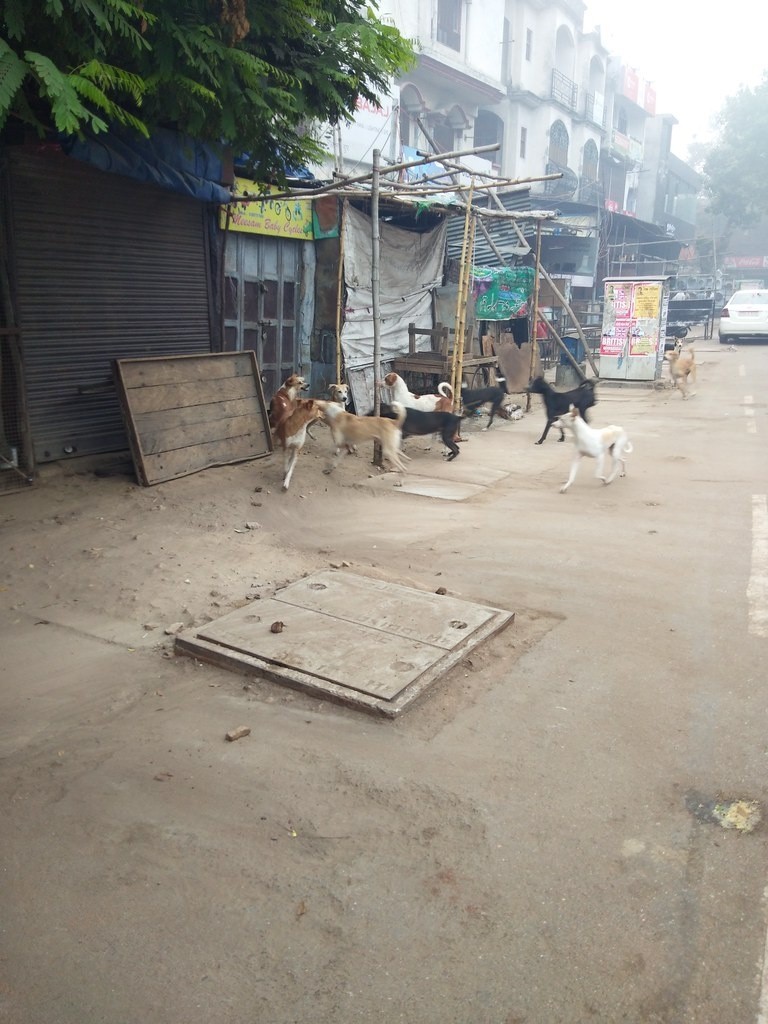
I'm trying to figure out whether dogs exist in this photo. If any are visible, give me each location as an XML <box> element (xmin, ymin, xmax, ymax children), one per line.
<box><xmin>461</xmin><ymin>386</ymin><xmax>504</xmax><ymax>430</ymax></box>
<box><xmin>552</xmin><ymin>407</ymin><xmax>633</xmax><ymax>493</ymax></box>
<box><xmin>313</xmin><ymin>399</ymin><xmax>413</xmax><ymax>487</ymax></box>
<box><xmin>269</xmin><ymin>374</ymin><xmax>358</xmax><ymax>489</ymax></box>
<box><xmin>524</xmin><ymin>376</ymin><xmax>598</xmax><ymax>444</ymax></box>
<box><xmin>378</xmin><ymin>371</ymin><xmax>454</xmax><ymax>454</ymax></box>
<box><xmin>664</xmin><ymin>337</ymin><xmax>697</xmax><ymax>399</ymax></box>
<box><xmin>364</xmin><ymin>403</ymin><xmax>460</xmax><ymax>462</ymax></box>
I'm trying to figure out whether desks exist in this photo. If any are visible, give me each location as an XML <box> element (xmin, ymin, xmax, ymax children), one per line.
<box><xmin>395</xmin><ymin>356</ymin><xmax>499</xmax><ymax>398</ymax></box>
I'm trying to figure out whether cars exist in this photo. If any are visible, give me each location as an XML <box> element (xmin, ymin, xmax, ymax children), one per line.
<box><xmin>718</xmin><ymin>288</ymin><xmax>768</xmax><ymax>345</ymax></box>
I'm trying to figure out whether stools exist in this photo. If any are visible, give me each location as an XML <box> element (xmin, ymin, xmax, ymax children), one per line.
<box><xmin>407</xmin><ymin>323</ymin><xmax>474</xmax><ymax>362</ymax></box>
